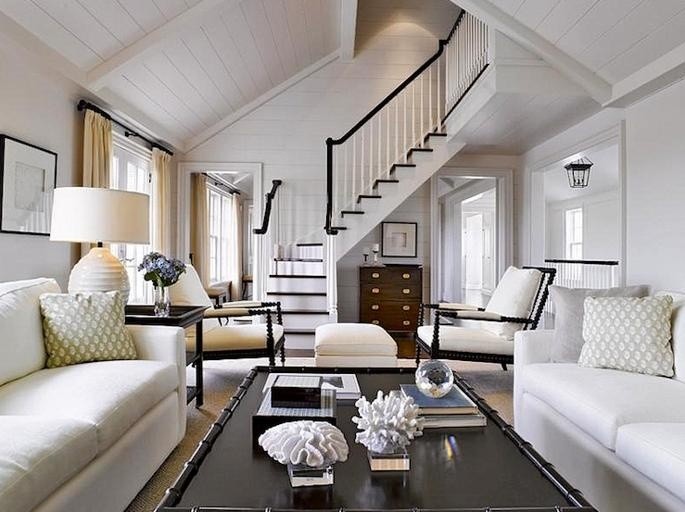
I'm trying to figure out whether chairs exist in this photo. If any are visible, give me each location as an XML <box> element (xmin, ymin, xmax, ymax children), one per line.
<box><xmin>415</xmin><ymin>266</ymin><xmax>557</xmax><ymax>368</ymax></box>
<box><xmin>186</xmin><ymin>301</ymin><xmax>286</xmax><ymax>373</ymax></box>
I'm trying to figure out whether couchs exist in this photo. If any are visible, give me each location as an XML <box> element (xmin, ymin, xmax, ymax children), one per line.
<box><xmin>510</xmin><ymin>290</ymin><xmax>685</xmax><ymax>512</ymax></box>
<box><xmin>0</xmin><ymin>277</ymin><xmax>187</xmax><ymax>512</ymax></box>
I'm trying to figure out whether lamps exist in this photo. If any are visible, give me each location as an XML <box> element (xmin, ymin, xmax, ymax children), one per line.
<box><xmin>564</xmin><ymin>157</ymin><xmax>594</xmax><ymax>188</ymax></box>
<box><xmin>49</xmin><ymin>187</ymin><xmax>149</xmax><ymax>305</ymax></box>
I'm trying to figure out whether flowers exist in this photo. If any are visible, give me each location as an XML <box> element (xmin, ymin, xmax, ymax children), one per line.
<box><xmin>137</xmin><ymin>252</ymin><xmax>186</xmax><ymax>311</ymax></box>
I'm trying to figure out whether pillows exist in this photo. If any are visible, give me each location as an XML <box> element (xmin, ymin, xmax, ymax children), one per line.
<box><xmin>546</xmin><ymin>284</ymin><xmax>648</xmax><ymax>363</ymax></box>
<box><xmin>578</xmin><ymin>294</ymin><xmax>674</xmax><ymax>378</ymax></box>
<box><xmin>41</xmin><ymin>291</ymin><xmax>138</xmax><ymax>368</ymax></box>
<box><xmin>168</xmin><ymin>263</ymin><xmax>218</xmax><ymax>334</ymax></box>
<box><xmin>481</xmin><ymin>265</ymin><xmax>543</xmax><ymax>341</ymax></box>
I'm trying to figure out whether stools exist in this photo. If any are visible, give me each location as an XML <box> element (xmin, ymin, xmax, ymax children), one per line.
<box><xmin>314</xmin><ymin>323</ymin><xmax>398</xmax><ymax>368</ymax></box>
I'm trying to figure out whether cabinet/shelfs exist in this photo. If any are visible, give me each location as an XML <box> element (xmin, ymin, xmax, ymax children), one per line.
<box><xmin>357</xmin><ymin>264</ymin><xmax>422</xmax><ymax>334</ymax></box>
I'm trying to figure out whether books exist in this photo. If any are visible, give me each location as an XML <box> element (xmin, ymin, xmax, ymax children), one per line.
<box><xmin>262</xmin><ymin>371</ymin><xmax>362</xmax><ymax>401</ymax></box>
<box><xmin>400</xmin><ymin>383</ymin><xmax>479</xmax><ymax>417</ymax></box>
<box><xmin>413</xmin><ymin>409</ymin><xmax>487</xmax><ymax>429</ymax></box>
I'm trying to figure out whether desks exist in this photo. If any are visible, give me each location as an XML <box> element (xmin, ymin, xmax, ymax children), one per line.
<box><xmin>241</xmin><ymin>275</ymin><xmax>253</xmax><ymax>299</ymax></box>
<box><xmin>123</xmin><ymin>304</ymin><xmax>212</xmax><ymax>407</ymax></box>
<box><xmin>206</xmin><ymin>289</ymin><xmax>229</xmax><ymax>326</ymax></box>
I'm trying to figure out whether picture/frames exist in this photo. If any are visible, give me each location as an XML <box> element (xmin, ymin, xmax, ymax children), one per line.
<box><xmin>0</xmin><ymin>134</ymin><xmax>57</xmax><ymax>234</ymax></box>
<box><xmin>381</xmin><ymin>221</ymin><xmax>417</xmax><ymax>258</ymax></box>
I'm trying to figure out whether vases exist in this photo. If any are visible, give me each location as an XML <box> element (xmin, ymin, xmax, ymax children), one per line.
<box><xmin>153</xmin><ymin>286</ymin><xmax>170</xmax><ymax>317</ymax></box>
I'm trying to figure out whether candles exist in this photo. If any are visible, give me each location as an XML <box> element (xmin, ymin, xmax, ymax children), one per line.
<box><xmin>363</xmin><ymin>246</ymin><xmax>370</xmax><ymax>255</ymax></box>
<box><xmin>373</xmin><ymin>243</ymin><xmax>379</xmax><ymax>252</ymax></box>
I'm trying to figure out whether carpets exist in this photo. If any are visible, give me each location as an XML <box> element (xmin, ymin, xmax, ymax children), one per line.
<box><xmin>126</xmin><ymin>389</ymin><xmax>514</xmax><ymax>512</ymax></box>
